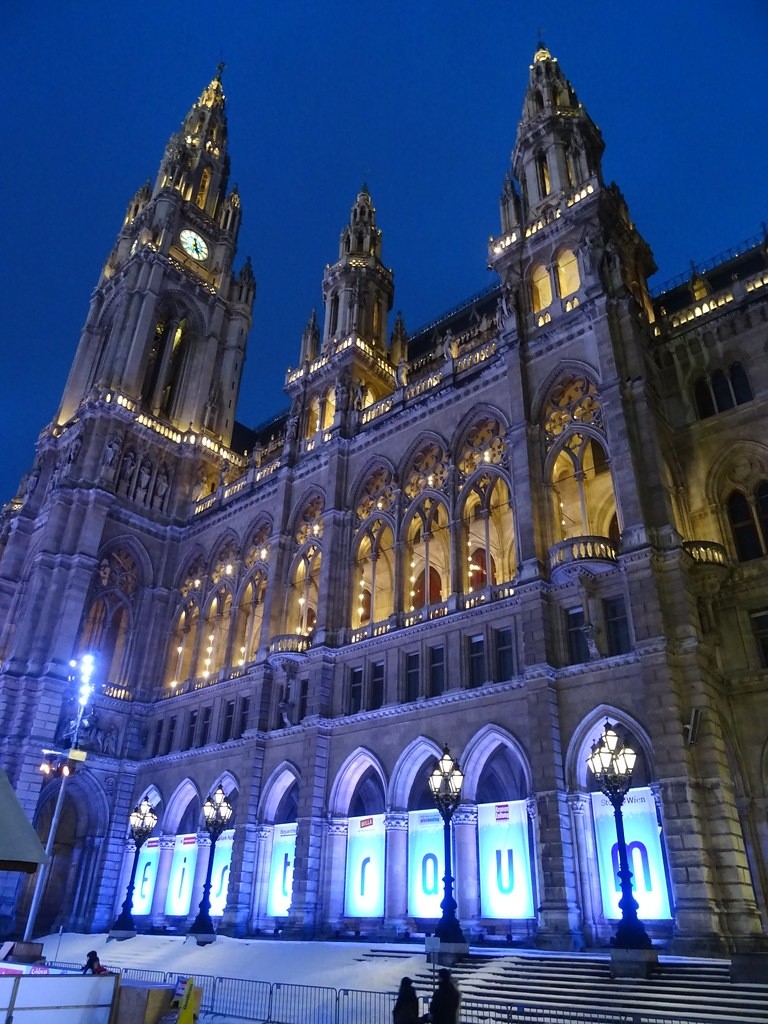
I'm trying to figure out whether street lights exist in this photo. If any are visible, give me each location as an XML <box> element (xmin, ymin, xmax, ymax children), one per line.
<box><xmin>102</xmin><ymin>796</ymin><xmax>156</xmax><ymax>944</ymax></box>
<box><xmin>182</xmin><ymin>783</ymin><xmax>234</xmax><ymax>947</ymax></box>
<box><xmin>584</xmin><ymin>714</ymin><xmax>659</xmax><ymax>976</ymax></box>
<box><xmin>5</xmin><ymin>655</ymin><xmax>100</xmax><ymax>942</ymax></box>
<box><xmin>425</xmin><ymin>742</ymin><xmax>471</xmax><ymax>965</ymax></box>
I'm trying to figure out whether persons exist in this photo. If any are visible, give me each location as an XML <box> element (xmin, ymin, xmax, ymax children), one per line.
<box><xmin>51</xmin><ymin>435</ymin><xmax>169</xmax><ymax>510</ymax></box>
<box><xmin>443</xmin><ymin>328</ymin><xmax>455</xmax><ymax>360</ymax></box>
<box><xmin>83</xmin><ymin>950</ymin><xmax>113</xmax><ymax>976</ymax></box>
<box><xmin>392</xmin><ymin>977</ymin><xmax>419</xmax><ymax>1024</ymax></box>
<box><xmin>396</xmin><ymin>356</ymin><xmax>410</xmax><ymax>386</ymax></box>
<box><xmin>495</xmin><ymin>298</ymin><xmax>506</xmax><ymax>330</ymax></box>
<box><xmin>428</xmin><ymin>968</ymin><xmax>460</xmax><ymax>1024</ymax></box>
<box><xmin>352</xmin><ymin>378</ymin><xmax>363</xmax><ymax>409</ymax></box>
<box><xmin>192</xmin><ymin>442</ymin><xmax>263</xmax><ymax>500</ymax></box>
<box><xmin>579</xmin><ymin>235</ymin><xmax>596</xmax><ymax>275</ymax></box>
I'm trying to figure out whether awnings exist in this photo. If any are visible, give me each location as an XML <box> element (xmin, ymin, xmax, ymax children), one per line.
<box><xmin>0</xmin><ymin>771</ymin><xmax>49</xmax><ymax>874</ymax></box>
